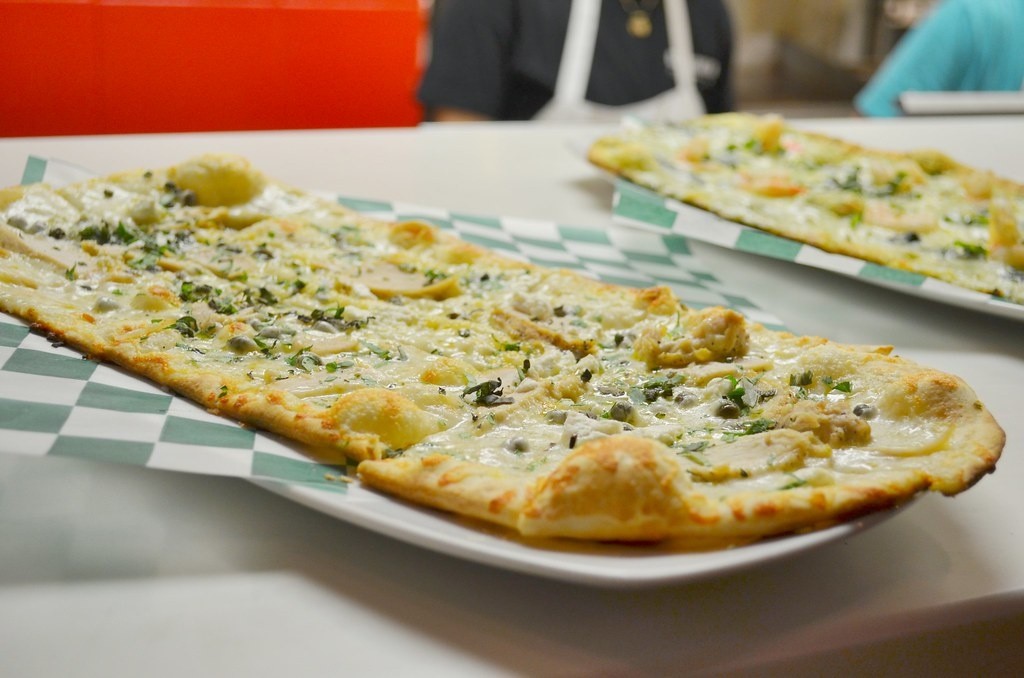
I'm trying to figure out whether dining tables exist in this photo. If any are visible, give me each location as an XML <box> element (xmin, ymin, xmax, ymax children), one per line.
<box><xmin>2</xmin><ymin>117</ymin><xmax>1024</xmax><ymax>675</ymax></box>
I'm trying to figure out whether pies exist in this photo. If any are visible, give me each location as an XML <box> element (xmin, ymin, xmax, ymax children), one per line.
<box><xmin>586</xmin><ymin>110</ymin><xmax>1024</xmax><ymax>306</ymax></box>
<box><xmin>1</xmin><ymin>154</ymin><xmax>1006</xmax><ymax>541</ymax></box>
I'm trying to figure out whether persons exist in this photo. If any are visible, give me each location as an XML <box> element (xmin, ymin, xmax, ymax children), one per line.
<box><xmin>416</xmin><ymin>0</ymin><xmax>737</xmax><ymax>123</ymax></box>
<box><xmin>851</xmin><ymin>1</ymin><xmax>1024</xmax><ymax>117</ymax></box>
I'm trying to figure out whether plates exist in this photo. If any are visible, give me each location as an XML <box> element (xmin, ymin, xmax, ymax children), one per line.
<box><xmin>568</xmin><ymin>130</ymin><xmax>1024</xmax><ymax>324</ymax></box>
<box><xmin>246</xmin><ymin>466</ymin><xmax>917</xmax><ymax>587</ymax></box>
